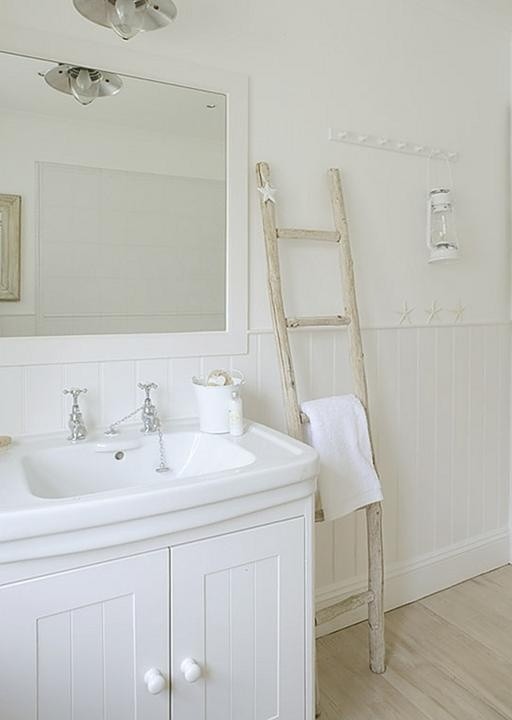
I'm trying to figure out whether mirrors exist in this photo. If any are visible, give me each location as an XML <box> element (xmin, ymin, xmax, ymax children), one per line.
<box><xmin>1</xmin><ymin>52</ymin><xmax>229</xmax><ymax>338</ymax></box>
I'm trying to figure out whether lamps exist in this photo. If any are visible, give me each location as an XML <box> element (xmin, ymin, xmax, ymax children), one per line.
<box><xmin>44</xmin><ymin>63</ymin><xmax>124</xmax><ymax>106</ymax></box>
<box><xmin>73</xmin><ymin>1</ymin><xmax>177</xmax><ymax>41</ymax></box>
<box><xmin>424</xmin><ymin>186</ymin><xmax>462</xmax><ymax>263</ymax></box>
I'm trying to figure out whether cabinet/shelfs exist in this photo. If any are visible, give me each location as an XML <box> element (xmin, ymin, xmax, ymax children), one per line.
<box><xmin>0</xmin><ymin>494</ymin><xmax>316</xmax><ymax>719</ymax></box>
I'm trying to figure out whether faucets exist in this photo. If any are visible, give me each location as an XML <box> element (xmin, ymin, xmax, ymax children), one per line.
<box><xmin>137</xmin><ymin>379</ymin><xmax>161</xmax><ymax>434</ymax></box>
<box><xmin>61</xmin><ymin>387</ymin><xmax>89</xmax><ymax>443</ymax></box>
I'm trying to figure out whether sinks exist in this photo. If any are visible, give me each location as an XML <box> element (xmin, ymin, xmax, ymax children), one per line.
<box><xmin>19</xmin><ymin>428</ymin><xmax>258</xmax><ymax>503</ymax></box>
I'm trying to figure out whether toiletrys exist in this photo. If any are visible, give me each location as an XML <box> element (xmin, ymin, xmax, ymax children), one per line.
<box><xmin>228</xmin><ymin>390</ymin><xmax>243</xmax><ymax>436</ymax></box>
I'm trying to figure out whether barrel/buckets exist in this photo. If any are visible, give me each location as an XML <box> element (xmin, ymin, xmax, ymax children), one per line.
<box><xmin>192</xmin><ymin>368</ymin><xmax>246</xmax><ymax>434</ymax></box>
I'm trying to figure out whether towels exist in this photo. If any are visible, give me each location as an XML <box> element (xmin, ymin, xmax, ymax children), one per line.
<box><xmin>298</xmin><ymin>393</ymin><xmax>384</xmax><ymax>525</ymax></box>
